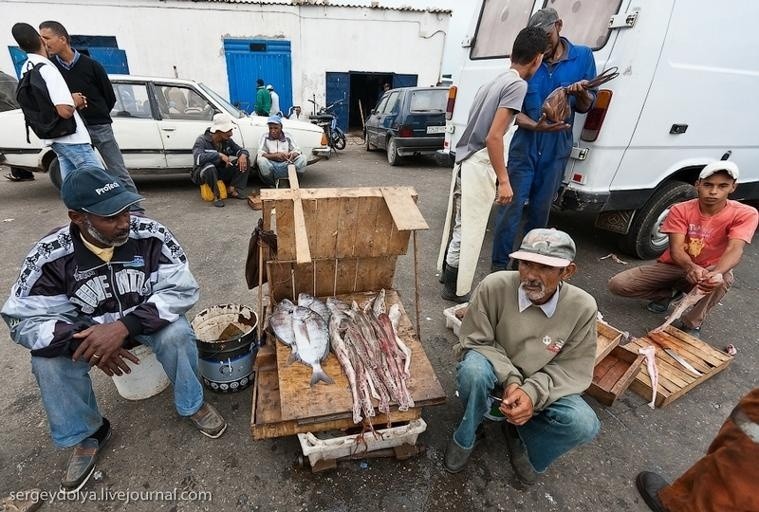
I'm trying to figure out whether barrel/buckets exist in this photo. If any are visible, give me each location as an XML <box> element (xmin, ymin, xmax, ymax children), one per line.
<box><xmin>190</xmin><ymin>302</ymin><xmax>258</xmax><ymax>394</ymax></box>
<box><xmin>111</xmin><ymin>344</ymin><xmax>172</xmax><ymax>401</ymax></box>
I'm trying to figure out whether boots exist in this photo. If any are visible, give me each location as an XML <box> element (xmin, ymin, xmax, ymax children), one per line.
<box><xmin>439</xmin><ymin>261</ymin><xmax>469</xmax><ymax>304</ymax></box>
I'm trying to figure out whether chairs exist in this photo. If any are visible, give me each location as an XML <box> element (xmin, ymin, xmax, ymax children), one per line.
<box><xmin>167</xmin><ymin>87</ymin><xmax>187</xmax><ymax>115</ymax></box>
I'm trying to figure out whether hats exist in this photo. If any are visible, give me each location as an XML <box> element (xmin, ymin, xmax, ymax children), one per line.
<box><xmin>528</xmin><ymin>8</ymin><xmax>559</xmax><ymax>33</ymax></box>
<box><xmin>267</xmin><ymin>116</ymin><xmax>281</xmax><ymax>124</ymax></box>
<box><xmin>210</xmin><ymin>113</ymin><xmax>236</xmax><ymax>134</ymax></box>
<box><xmin>698</xmin><ymin>160</ymin><xmax>739</xmax><ymax>181</ymax></box>
<box><xmin>508</xmin><ymin>228</ymin><xmax>576</xmax><ymax>267</ymax></box>
<box><xmin>60</xmin><ymin>165</ymin><xmax>146</xmax><ymax>217</ymax></box>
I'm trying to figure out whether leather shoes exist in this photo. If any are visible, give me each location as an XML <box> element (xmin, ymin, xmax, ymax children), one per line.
<box><xmin>227</xmin><ymin>189</ymin><xmax>245</xmax><ymax>199</ymax></box>
<box><xmin>59</xmin><ymin>429</ymin><xmax>112</xmax><ymax>494</ymax></box>
<box><xmin>647</xmin><ymin>290</ymin><xmax>683</xmax><ymax>313</ymax></box>
<box><xmin>442</xmin><ymin>434</ymin><xmax>473</xmax><ymax>473</ymax></box>
<box><xmin>189</xmin><ymin>401</ymin><xmax>227</xmax><ymax>439</ymax></box>
<box><xmin>636</xmin><ymin>471</ymin><xmax>671</xmax><ymax>512</ymax></box>
<box><xmin>680</xmin><ymin>323</ymin><xmax>702</xmax><ymax>342</ymax></box>
<box><xmin>214</xmin><ymin>197</ymin><xmax>224</xmax><ymax>207</ymax></box>
<box><xmin>502</xmin><ymin>420</ymin><xmax>536</xmax><ymax>484</ymax></box>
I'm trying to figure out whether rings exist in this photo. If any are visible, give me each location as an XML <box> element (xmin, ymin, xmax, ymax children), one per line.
<box><xmin>92</xmin><ymin>353</ymin><xmax>103</xmax><ymax>361</ymax></box>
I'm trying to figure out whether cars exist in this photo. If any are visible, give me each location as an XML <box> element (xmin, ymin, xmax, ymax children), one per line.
<box><xmin>0</xmin><ymin>74</ymin><xmax>331</xmax><ymax>195</ymax></box>
<box><xmin>363</xmin><ymin>86</ymin><xmax>451</xmax><ymax>167</ymax></box>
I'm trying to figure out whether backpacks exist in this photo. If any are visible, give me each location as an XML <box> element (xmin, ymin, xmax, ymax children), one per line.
<box><xmin>15</xmin><ymin>61</ymin><xmax>77</xmax><ymax>139</ymax></box>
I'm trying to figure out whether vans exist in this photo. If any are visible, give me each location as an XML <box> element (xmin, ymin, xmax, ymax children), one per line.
<box><xmin>432</xmin><ymin>0</ymin><xmax>759</xmax><ymax>259</ymax></box>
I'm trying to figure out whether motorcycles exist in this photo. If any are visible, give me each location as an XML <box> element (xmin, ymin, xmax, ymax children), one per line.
<box><xmin>305</xmin><ymin>98</ymin><xmax>345</xmax><ymax>157</ymax></box>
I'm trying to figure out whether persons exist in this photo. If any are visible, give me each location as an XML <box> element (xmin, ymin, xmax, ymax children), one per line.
<box><xmin>634</xmin><ymin>387</ymin><xmax>759</xmax><ymax>512</ymax></box>
<box><xmin>256</xmin><ymin>115</ymin><xmax>307</xmax><ymax>188</ymax></box>
<box><xmin>438</xmin><ymin>225</ymin><xmax>602</xmax><ymax>490</ymax></box>
<box><xmin>378</xmin><ymin>80</ymin><xmax>393</xmax><ymax>106</ymax></box>
<box><xmin>487</xmin><ymin>6</ymin><xmax>598</xmax><ymax>274</ymax></box>
<box><xmin>606</xmin><ymin>160</ymin><xmax>759</xmax><ymax>338</ymax></box>
<box><xmin>433</xmin><ymin>24</ymin><xmax>548</xmax><ymax>305</ymax></box>
<box><xmin>188</xmin><ymin>112</ymin><xmax>251</xmax><ymax>208</ymax></box>
<box><xmin>10</xmin><ymin>22</ymin><xmax>105</xmax><ymax>184</ymax></box>
<box><xmin>39</xmin><ymin>19</ymin><xmax>139</xmax><ymax>209</ymax></box>
<box><xmin>160</xmin><ymin>86</ymin><xmax>209</xmax><ymax>118</ymax></box>
<box><xmin>1</xmin><ymin>163</ymin><xmax>227</xmax><ymax>498</ymax></box>
<box><xmin>253</xmin><ymin>77</ymin><xmax>308</xmax><ymax>120</ymax></box>
